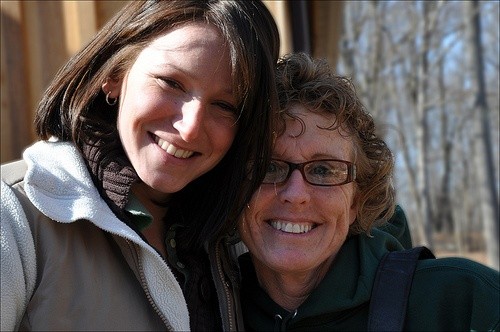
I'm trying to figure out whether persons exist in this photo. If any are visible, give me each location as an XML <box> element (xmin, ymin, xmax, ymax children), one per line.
<box><xmin>1</xmin><ymin>0</ymin><xmax>280</xmax><ymax>332</ymax></box>
<box><xmin>232</xmin><ymin>52</ymin><xmax>500</xmax><ymax>332</ymax></box>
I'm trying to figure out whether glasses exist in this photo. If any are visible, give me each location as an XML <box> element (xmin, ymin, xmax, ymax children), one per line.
<box><xmin>243</xmin><ymin>157</ymin><xmax>357</xmax><ymax>187</ymax></box>
